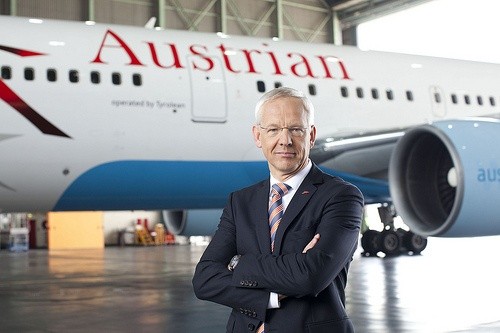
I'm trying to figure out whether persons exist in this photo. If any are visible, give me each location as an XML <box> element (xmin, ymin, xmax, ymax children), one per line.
<box><xmin>193</xmin><ymin>86</ymin><xmax>363</xmax><ymax>333</ymax></box>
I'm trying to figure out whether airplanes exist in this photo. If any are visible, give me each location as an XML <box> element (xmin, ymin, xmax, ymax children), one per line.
<box><xmin>2</xmin><ymin>9</ymin><xmax>499</xmax><ymax>260</ymax></box>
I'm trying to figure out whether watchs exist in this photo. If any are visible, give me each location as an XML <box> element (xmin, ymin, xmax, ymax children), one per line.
<box><xmin>230</xmin><ymin>254</ymin><xmax>241</xmax><ymax>271</ymax></box>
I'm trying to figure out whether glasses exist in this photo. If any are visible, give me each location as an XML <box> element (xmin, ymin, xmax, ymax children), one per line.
<box><xmin>258</xmin><ymin>123</ymin><xmax>311</xmax><ymax>137</ymax></box>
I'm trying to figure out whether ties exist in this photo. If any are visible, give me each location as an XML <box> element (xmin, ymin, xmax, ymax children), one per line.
<box><xmin>268</xmin><ymin>183</ymin><xmax>292</xmax><ymax>253</ymax></box>
